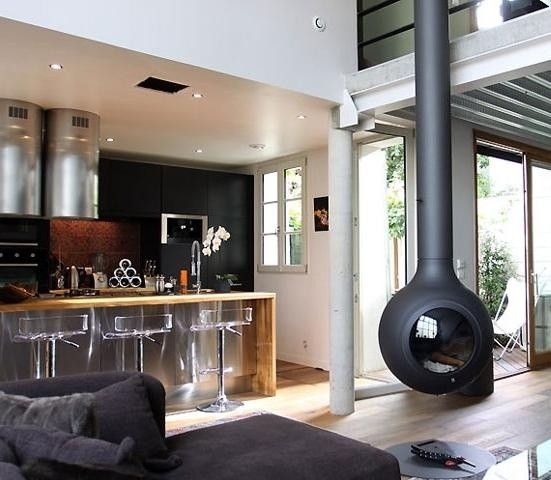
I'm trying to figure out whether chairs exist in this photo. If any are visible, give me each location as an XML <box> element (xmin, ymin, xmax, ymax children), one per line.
<box><xmin>101</xmin><ymin>314</ymin><xmax>173</xmax><ymax>373</ymax></box>
<box><xmin>7</xmin><ymin>314</ymin><xmax>89</xmax><ymax>379</ymax></box>
<box><xmin>491</xmin><ymin>277</ymin><xmax>547</xmax><ymax>361</ymax></box>
<box><xmin>190</xmin><ymin>307</ymin><xmax>253</xmax><ymax>413</ymax></box>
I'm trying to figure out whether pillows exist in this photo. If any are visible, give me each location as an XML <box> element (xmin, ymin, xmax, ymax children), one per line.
<box><xmin>19</xmin><ymin>457</ymin><xmax>160</xmax><ymax>480</ymax></box>
<box><xmin>0</xmin><ymin>425</ymin><xmax>151</xmax><ymax>476</ymax></box>
<box><xmin>93</xmin><ymin>374</ymin><xmax>183</xmax><ymax>474</ymax></box>
<box><xmin>0</xmin><ymin>390</ymin><xmax>96</xmax><ymax>438</ymax></box>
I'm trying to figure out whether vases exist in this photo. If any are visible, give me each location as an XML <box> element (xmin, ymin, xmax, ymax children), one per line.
<box><xmin>214</xmin><ymin>280</ymin><xmax>231</xmax><ymax>293</ymax></box>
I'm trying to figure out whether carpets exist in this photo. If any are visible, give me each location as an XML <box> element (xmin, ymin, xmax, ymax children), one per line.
<box><xmin>408</xmin><ymin>446</ymin><xmax>523</xmax><ymax>480</ymax></box>
<box><xmin>384</xmin><ymin>440</ymin><xmax>496</xmax><ymax>480</ymax></box>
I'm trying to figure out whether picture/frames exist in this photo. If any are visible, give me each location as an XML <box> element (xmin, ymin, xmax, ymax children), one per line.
<box><xmin>312</xmin><ymin>195</ymin><xmax>330</xmax><ymax>233</ymax></box>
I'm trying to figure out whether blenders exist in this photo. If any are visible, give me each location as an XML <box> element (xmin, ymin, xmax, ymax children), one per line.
<box><xmin>91</xmin><ymin>254</ymin><xmax>108</xmax><ymax>288</ymax></box>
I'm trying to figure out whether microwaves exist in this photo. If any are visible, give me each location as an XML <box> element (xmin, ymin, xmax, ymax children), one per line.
<box><xmin>162</xmin><ymin>214</ymin><xmax>207</xmax><ymax>246</ymax></box>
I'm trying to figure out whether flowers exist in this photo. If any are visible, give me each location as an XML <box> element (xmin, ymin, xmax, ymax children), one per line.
<box><xmin>202</xmin><ymin>225</ymin><xmax>231</xmax><ymax>259</ymax></box>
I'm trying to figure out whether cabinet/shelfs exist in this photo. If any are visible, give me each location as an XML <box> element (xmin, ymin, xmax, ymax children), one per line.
<box><xmin>162</xmin><ymin>165</ymin><xmax>208</xmax><ymax>215</ymax></box>
<box><xmin>99</xmin><ymin>158</ymin><xmax>162</xmax><ymax>218</ymax></box>
<box><xmin>208</xmin><ymin>170</ymin><xmax>254</xmax><ymax>292</ymax></box>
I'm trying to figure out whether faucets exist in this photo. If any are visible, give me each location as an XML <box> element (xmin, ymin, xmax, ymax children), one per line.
<box><xmin>191</xmin><ymin>240</ymin><xmax>201</xmax><ymax>294</ymax></box>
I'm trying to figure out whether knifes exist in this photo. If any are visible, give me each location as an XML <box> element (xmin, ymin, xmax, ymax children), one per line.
<box><xmin>143</xmin><ymin>258</ymin><xmax>156</xmax><ymax>276</ymax></box>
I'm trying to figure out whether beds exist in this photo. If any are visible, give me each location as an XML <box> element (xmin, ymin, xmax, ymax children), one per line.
<box><xmin>0</xmin><ymin>371</ymin><xmax>401</xmax><ymax>480</ymax></box>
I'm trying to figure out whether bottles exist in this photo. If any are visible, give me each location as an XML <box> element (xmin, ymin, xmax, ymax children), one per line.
<box><xmin>85</xmin><ymin>267</ymin><xmax>93</xmax><ymax>287</ymax></box>
<box><xmin>155</xmin><ymin>273</ymin><xmax>164</xmax><ymax>294</ymax></box>
<box><xmin>64</xmin><ymin>266</ymin><xmax>70</xmax><ymax>288</ymax></box>
<box><xmin>71</xmin><ymin>265</ymin><xmax>78</xmax><ymax>288</ymax></box>
<box><xmin>180</xmin><ymin>269</ymin><xmax>187</xmax><ymax>293</ymax></box>
<box><xmin>77</xmin><ymin>267</ymin><xmax>85</xmax><ymax>287</ymax></box>
